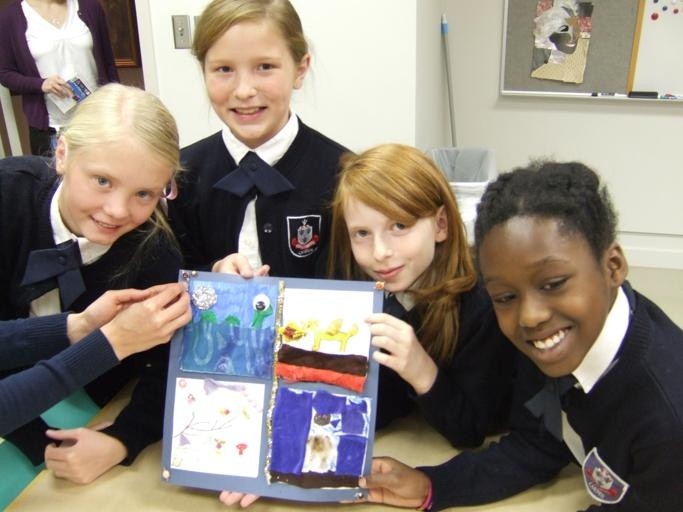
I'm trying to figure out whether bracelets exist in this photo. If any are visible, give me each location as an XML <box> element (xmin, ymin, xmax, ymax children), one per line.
<box><xmin>414</xmin><ymin>476</ymin><xmax>434</xmax><ymax>512</ymax></box>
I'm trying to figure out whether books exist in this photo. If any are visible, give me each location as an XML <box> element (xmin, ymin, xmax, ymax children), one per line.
<box><xmin>44</xmin><ymin>73</ymin><xmax>91</xmax><ymax>114</ymax></box>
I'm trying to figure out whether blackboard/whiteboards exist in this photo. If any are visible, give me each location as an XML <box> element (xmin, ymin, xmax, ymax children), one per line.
<box><xmin>499</xmin><ymin>0</ymin><xmax>683</xmax><ymax>103</ymax></box>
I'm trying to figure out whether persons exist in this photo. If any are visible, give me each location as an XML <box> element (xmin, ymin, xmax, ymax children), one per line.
<box><xmin>164</xmin><ymin>0</ymin><xmax>364</xmax><ymax>285</ymax></box>
<box><xmin>351</xmin><ymin>160</ymin><xmax>683</xmax><ymax>512</ymax></box>
<box><xmin>0</xmin><ymin>280</ymin><xmax>196</xmax><ymax>437</ymax></box>
<box><xmin>0</xmin><ymin>82</ymin><xmax>188</xmax><ymax>484</ymax></box>
<box><xmin>214</xmin><ymin>143</ymin><xmax>515</xmax><ymax>504</ymax></box>
<box><xmin>0</xmin><ymin>0</ymin><xmax>121</xmax><ymax>158</ymax></box>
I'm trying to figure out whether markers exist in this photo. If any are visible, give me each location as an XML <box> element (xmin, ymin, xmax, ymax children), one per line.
<box><xmin>592</xmin><ymin>92</ymin><xmax>618</xmax><ymax>96</ymax></box>
<box><xmin>660</xmin><ymin>96</ymin><xmax>681</xmax><ymax>99</ymax></box>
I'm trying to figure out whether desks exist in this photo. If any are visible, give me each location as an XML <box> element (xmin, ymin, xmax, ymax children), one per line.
<box><xmin>2</xmin><ymin>376</ymin><xmax>601</xmax><ymax>512</ymax></box>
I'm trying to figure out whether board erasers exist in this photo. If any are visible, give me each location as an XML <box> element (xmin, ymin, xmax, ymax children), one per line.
<box><xmin>628</xmin><ymin>92</ymin><xmax>659</xmax><ymax>98</ymax></box>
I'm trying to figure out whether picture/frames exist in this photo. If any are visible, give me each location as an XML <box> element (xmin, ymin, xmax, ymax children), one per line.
<box><xmin>100</xmin><ymin>0</ymin><xmax>141</xmax><ymax>70</ymax></box>
